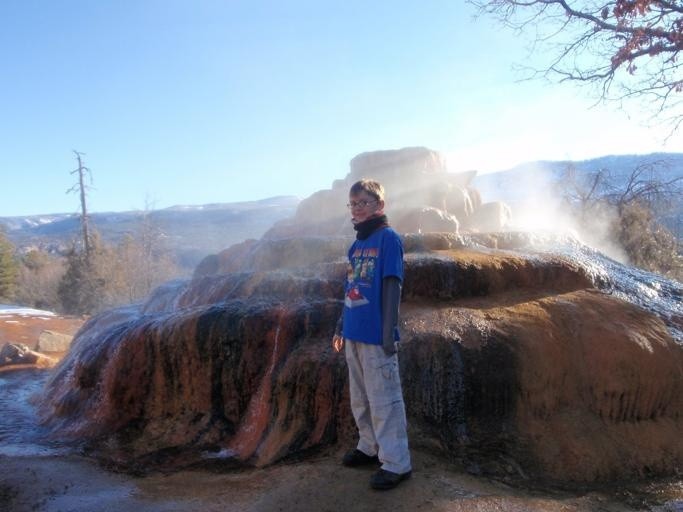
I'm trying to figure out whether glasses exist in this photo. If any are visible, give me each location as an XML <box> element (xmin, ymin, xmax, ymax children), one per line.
<box><xmin>346</xmin><ymin>199</ymin><xmax>379</xmax><ymax>209</ymax></box>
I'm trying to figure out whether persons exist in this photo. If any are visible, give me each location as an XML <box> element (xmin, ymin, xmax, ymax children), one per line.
<box><xmin>329</xmin><ymin>177</ymin><xmax>418</xmax><ymax>492</ymax></box>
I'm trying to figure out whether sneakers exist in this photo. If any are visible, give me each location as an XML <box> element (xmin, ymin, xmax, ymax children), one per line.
<box><xmin>343</xmin><ymin>448</ymin><xmax>378</xmax><ymax>466</ymax></box>
<box><xmin>370</xmin><ymin>468</ymin><xmax>411</xmax><ymax>490</ymax></box>
